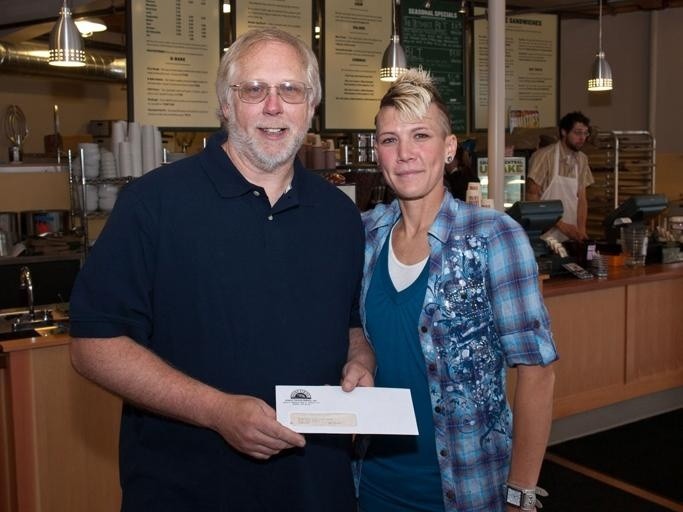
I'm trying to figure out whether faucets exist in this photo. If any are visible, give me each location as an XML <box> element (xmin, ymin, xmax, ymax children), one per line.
<box><xmin>20</xmin><ymin>266</ymin><xmax>35</xmax><ymax>311</ymax></box>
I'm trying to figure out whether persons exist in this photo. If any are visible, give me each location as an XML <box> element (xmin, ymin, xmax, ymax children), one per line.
<box><xmin>525</xmin><ymin>109</ymin><xmax>595</xmax><ymax>244</ymax></box>
<box><xmin>442</xmin><ymin>133</ymin><xmax>558</xmax><ymax>203</ymax></box>
<box><xmin>356</xmin><ymin>66</ymin><xmax>559</xmax><ymax>512</ymax></box>
<box><xmin>66</xmin><ymin>26</ymin><xmax>377</xmax><ymax>510</ymax></box>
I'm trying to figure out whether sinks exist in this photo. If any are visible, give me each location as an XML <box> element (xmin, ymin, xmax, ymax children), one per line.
<box><xmin>1</xmin><ymin>310</ymin><xmax>53</xmax><ymax>328</ymax></box>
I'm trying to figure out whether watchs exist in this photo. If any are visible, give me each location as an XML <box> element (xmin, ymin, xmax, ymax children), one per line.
<box><xmin>499</xmin><ymin>476</ymin><xmax>548</xmax><ymax>511</ymax></box>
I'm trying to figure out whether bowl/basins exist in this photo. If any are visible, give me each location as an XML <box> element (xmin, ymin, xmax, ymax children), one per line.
<box><xmin>72</xmin><ymin>140</ymin><xmax>117</xmax><ymax>212</ymax></box>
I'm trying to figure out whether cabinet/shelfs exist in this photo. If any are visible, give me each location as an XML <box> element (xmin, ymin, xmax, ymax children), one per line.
<box><xmin>0</xmin><ymin>75</ymin><xmax>209</xmax><ymax>308</ymax></box>
<box><xmin>512</xmin><ymin>127</ymin><xmax>657</xmax><ymax>239</ymax></box>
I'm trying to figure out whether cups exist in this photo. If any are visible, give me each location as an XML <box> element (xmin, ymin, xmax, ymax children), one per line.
<box><xmin>341</xmin><ymin>132</ymin><xmax>378</xmax><ymax>165</ymax></box>
<box><xmin>296</xmin><ymin>133</ymin><xmax>337</xmax><ymax>169</ymax></box>
<box><xmin>111</xmin><ymin>120</ymin><xmax>162</xmax><ymax>212</ymax></box>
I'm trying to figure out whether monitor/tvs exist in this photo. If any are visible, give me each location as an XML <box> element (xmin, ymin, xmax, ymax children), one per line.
<box><xmin>602</xmin><ymin>195</ymin><xmax>669</xmax><ymax>238</ymax></box>
<box><xmin>506</xmin><ymin>200</ymin><xmax>564</xmax><ymax>236</ymax></box>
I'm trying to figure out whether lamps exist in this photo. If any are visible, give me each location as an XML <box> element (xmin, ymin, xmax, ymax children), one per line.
<box><xmin>74</xmin><ymin>16</ymin><xmax>108</xmax><ymax>32</ymax></box>
<box><xmin>49</xmin><ymin>0</ymin><xmax>86</xmax><ymax>67</ymax></box>
<box><xmin>587</xmin><ymin>0</ymin><xmax>613</xmax><ymax>91</ymax></box>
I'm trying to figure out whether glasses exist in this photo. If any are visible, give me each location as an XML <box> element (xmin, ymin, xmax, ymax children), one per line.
<box><xmin>570</xmin><ymin>129</ymin><xmax>590</xmax><ymax>136</ymax></box>
<box><xmin>227</xmin><ymin>78</ymin><xmax>314</xmax><ymax>105</ymax></box>
